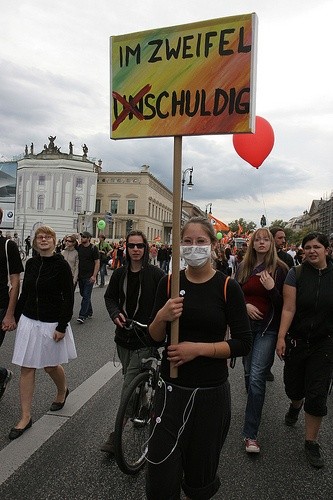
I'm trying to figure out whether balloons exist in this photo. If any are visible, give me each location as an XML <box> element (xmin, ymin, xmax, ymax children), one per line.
<box><xmin>233</xmin><ymin>116</ymin><xmax>274</xmax><ymax>169</ymax></box>
<box><xmin>97</xmin><ymin>220</ymin><xmax>105</xmax><ymax>230</ymax></box>
<box><xmin>217</xmin><ymin>232</ymin><xmax>222</xmax><ymax>240</ymax></box>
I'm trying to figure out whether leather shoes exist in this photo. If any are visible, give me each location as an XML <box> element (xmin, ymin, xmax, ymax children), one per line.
<box><xmin>8</xmin><ymin>417</ymin><xmax>32</xmax><ymax>440</ymax></box>
<box><xmin>49</xmin><ymin>388</ymin><xmax>70</xmax><ymax>411</ymax></box>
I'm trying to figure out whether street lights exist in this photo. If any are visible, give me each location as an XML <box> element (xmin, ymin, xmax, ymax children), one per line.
<box><xmin>180</xmin><ymin>166</ymin><xmax>194</xmax><ymax>225</ymax></box>
<box><xmin>205</xmin><ymin>202</ymin><xmax>213</xmax><ymax>219</ymax></box>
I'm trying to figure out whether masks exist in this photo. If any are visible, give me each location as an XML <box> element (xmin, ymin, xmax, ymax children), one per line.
<box><xmin>181</xmin><ymin>243</ymin><xmax>213</xmax><ymax>266</ymax></box>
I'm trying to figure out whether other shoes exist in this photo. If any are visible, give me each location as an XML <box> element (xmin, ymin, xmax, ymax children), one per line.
<box><xmin>285</xmin><ymin>397</ymin><xmax>303</xmax><ymax>425</ymax></box>
<box><xmin>244</xmin><ymin>436</ymin><xmax>260</xmax><ymax>453</ymax></box>
<box><xmin>304</xmin><ymin>440</ymin><xmax>325</xmax><ymax>469</ymax></box>
<box><xmin>100</xmin><ymin>428</ymin><xmax>121</xmax><ymax>454</ymax></box>
<box><xmin>0</xmin><ymin>369</ymin><xmax>13</xmax><ymax>398</ymax></box>
<box><xmin>265</xmin><ymin>371</ymin><xmax>274</xmax><ymax>381</ymax></box>
<box><xmin>133</xmin><ymin>404</ymin><xmax>151</xmax><ymax>423</ymax></box>
<box><xmin>76</xmin><ymin>311</ymin><xmax>93</xmax><ymax>324</ymax></box>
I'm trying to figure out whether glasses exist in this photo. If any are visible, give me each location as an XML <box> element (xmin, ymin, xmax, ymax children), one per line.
<box><xmin>81</xmin><ymin>235</ymin><xmax>88</xmax><ymax>238</ymax></box>
<box><xmin>125</xmin><ymin>241</ymin><xmax>144</xmax><ymax>250</ymax></box>
<box><xmin>33</xmin><ymin>235</ymin><xmax>51</xmax><ymax>241</ymax></box>
<box><xmin>65</xmin><ymin>240</ymin><xmax>73</xmax><ymax>243</ymax></box>
<box><xmin>178</xmin><ymin>237</ymin><xmax>210</xmax><ymax>248</ymax></box>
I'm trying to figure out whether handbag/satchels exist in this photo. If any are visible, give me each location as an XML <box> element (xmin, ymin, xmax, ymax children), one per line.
<box><xmin>100</xmin><ymin>248</ymin><xmax>113</xmax><ymax>263</ymax></box>
<box><xmin>5</xmin><ymin>239</ymin><xmax>25</xmax><ymax>300</ymax></box>
<box><xmin>281</xmin><ymin>331</ymin><xmax>308</xmax><ymax>372</ymax></box>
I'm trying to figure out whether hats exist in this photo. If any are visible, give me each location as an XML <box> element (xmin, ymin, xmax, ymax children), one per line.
<box><xmin>79</xmin><ymin>231</ymin><xmax>92</xmax><ymax>238</ymax></box>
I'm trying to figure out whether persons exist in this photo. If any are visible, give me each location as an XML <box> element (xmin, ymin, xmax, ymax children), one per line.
<box><xmin>0</xmin><ymin>209</ymin><xmax>333</xmax><ymax>500</ymax></box>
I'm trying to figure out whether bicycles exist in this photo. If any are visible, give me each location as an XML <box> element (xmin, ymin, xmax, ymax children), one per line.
<box><xmin>114</xmin><ymin>317</ymin><xmax>168</xmax><ymax>475</ymax></box>
